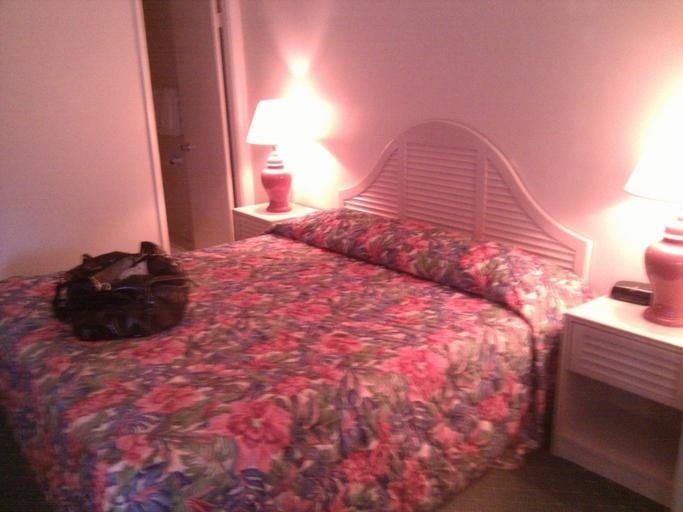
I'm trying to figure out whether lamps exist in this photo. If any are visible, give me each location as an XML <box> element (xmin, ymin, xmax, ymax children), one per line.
<box><xmin>624</xmin><ymin>133</ymin><xmax>682</xmax><ymax>328</ymax></box>
<box><xmin>245</xmin><ymin>100</ymin><xmax>297</xmax><ymax>213</ymax></box>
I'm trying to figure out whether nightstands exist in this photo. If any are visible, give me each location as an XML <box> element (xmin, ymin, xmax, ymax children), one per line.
<box><xmin>550</xmin><ymin>294</ymin><xmax>683</xmax><ymax>512</ymax></box>
<box><xmin>232</xmin><ymin>202</ymin><xmax>324</xmax><ymax>243</ymax></box>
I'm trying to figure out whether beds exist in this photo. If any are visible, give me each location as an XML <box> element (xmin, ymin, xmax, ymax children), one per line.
<box><xmin>0</xmin><ymin>118</ymin><xmax>594</xmax><ymax>512</ymax></box>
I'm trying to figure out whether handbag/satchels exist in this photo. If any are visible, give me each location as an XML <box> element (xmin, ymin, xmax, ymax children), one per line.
<box><xmin>52</xmin><ymin>242</ymin><xmax>189</xmax><ymax>342</ymax></box>
